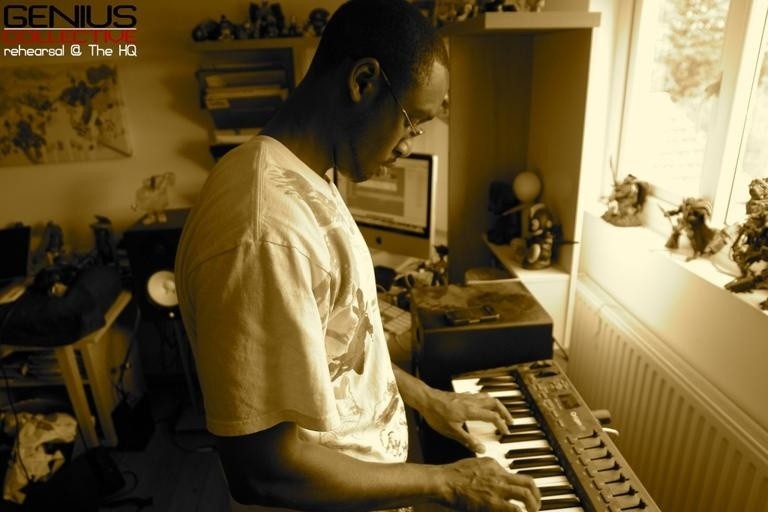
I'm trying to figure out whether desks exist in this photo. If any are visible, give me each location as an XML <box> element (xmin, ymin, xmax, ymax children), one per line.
<box><xmin>120</xmin><ymin>206</ymin><xmax>192</xmax><ymax>286</ymax></box>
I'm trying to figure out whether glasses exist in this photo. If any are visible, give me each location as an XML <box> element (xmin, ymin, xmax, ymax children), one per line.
<box><xmin>376</xmin><ymin>67</ymin><xmax>430</xmax><ymax>138</ymax></box>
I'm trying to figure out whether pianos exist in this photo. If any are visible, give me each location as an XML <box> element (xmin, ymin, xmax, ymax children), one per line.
<box><xmin>451</xmin><ymin>360</ymin><xmax>661</xmax><ymax>512</ymax></box>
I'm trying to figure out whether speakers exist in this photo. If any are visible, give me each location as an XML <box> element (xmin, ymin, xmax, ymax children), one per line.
<box><xmin>122</xmin><ymin>204</ymin><xmax>217</xmax><ymax>455</ymax></box>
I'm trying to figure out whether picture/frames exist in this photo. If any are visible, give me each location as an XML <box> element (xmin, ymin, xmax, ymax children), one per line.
<box><xmin>333</xmin><ymin>150</ymin><xmax>438</xmax><ymax>240</ymax></box>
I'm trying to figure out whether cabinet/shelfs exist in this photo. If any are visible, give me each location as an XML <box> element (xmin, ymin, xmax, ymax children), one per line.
<box><xmin>427</xmin><ymin>0</ymin><xmax>603</xmax><ymax>359</ymax></box>
<box><xmin>188</xmin><ymin>34</ymin><xmax>319</xmax><ymax>162</ymax></box>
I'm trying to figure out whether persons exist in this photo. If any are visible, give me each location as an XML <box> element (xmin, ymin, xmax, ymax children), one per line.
<box><xmin>523</xmin><ymin>203</ymin><xmax>559</xmax><ymax>272</ymax></box>
<box><xmin>176</xmin><ymin>0</ymin><xmax>543</xmax><ymax>512</ymax></box>
